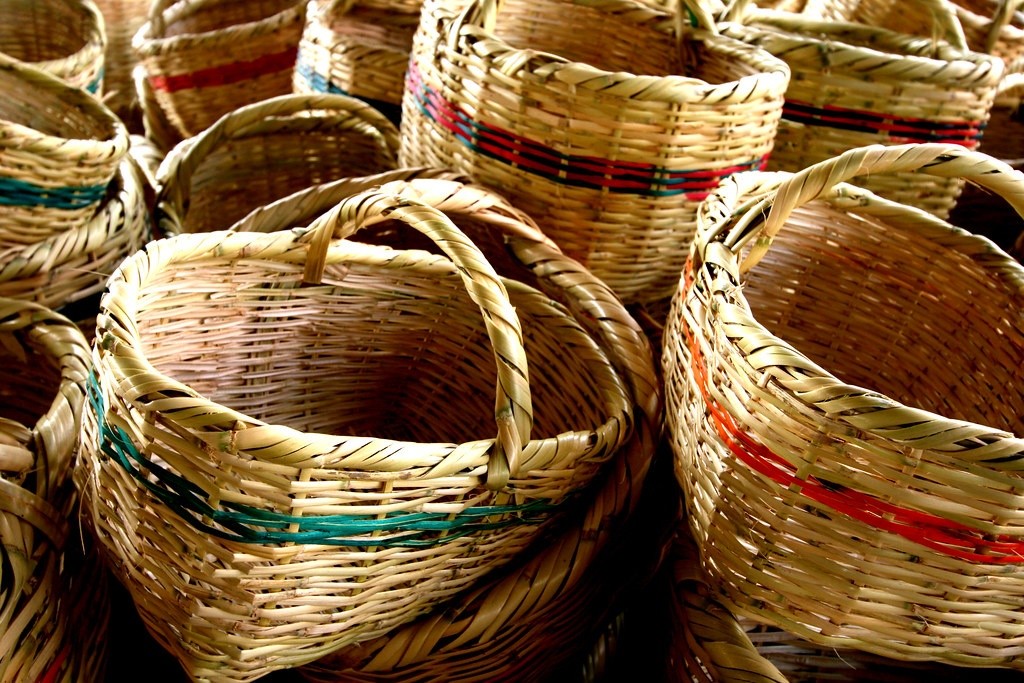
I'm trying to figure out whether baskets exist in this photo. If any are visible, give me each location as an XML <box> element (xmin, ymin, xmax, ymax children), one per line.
<box><xmin>0</xmin><ymin>0</ymin><xmax>1024</xmax><ymax>683</ymax></box>
